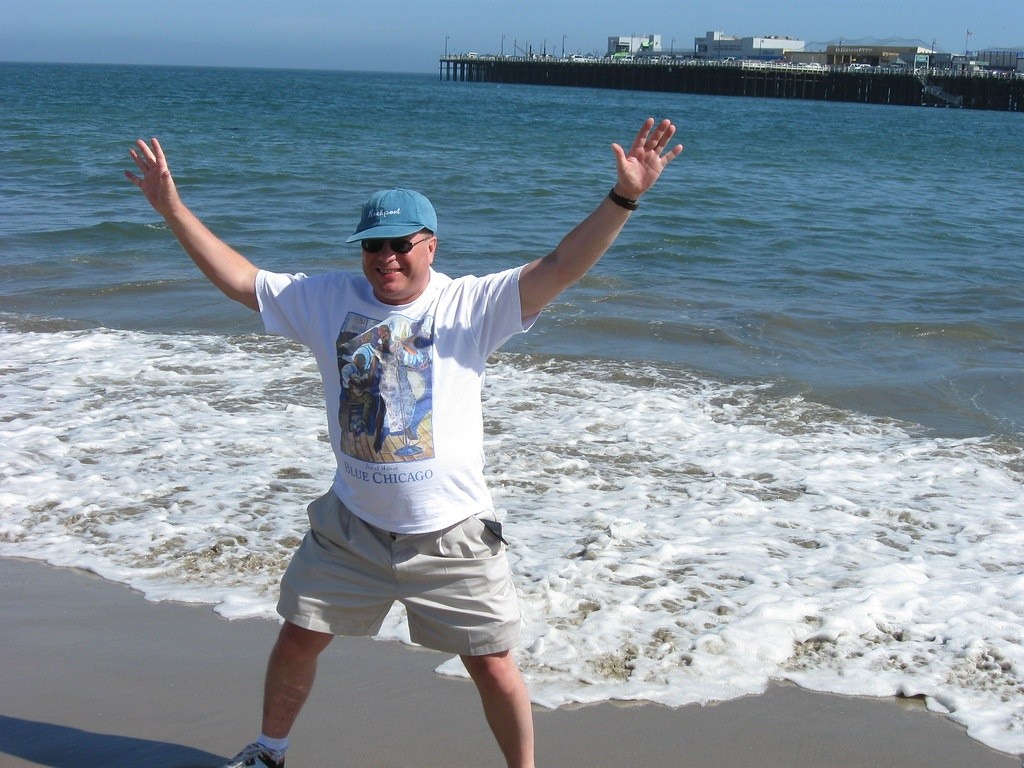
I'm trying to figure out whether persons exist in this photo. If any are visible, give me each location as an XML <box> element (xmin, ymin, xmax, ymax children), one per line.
<box><xmin>124</xmin><ymin>104</ymin><xmax>690</xmax><ymax>768</ymax></box>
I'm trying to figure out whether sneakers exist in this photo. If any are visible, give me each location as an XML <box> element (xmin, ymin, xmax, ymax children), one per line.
<box><xmin>223</xmin><ymin>742</ymin><xmax>285</xmax><ymax>768</ymax></box>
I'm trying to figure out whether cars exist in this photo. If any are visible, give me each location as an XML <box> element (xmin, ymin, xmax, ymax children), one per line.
<box><xmin>468</xmin><ymin>52</ymin><xmax>1024</xmax><ymax>80</ymax></box>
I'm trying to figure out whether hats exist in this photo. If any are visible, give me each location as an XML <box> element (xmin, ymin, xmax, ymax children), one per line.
<box><xmin>346</xmin><ymin>188</ymin><xmax>437</xmax><ymax>243</ymax></box>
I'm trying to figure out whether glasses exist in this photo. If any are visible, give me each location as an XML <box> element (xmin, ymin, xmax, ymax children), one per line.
<box><xmin>361</xmin><ymin>236</ymin><xmax>433</xmax><ymax>253</ymax></box>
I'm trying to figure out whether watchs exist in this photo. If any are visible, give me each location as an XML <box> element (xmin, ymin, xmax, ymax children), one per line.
<box><xmin>607</xmin><ymin>189</ymin><xmax>640</xmax><ymax>214</ymax></box>
